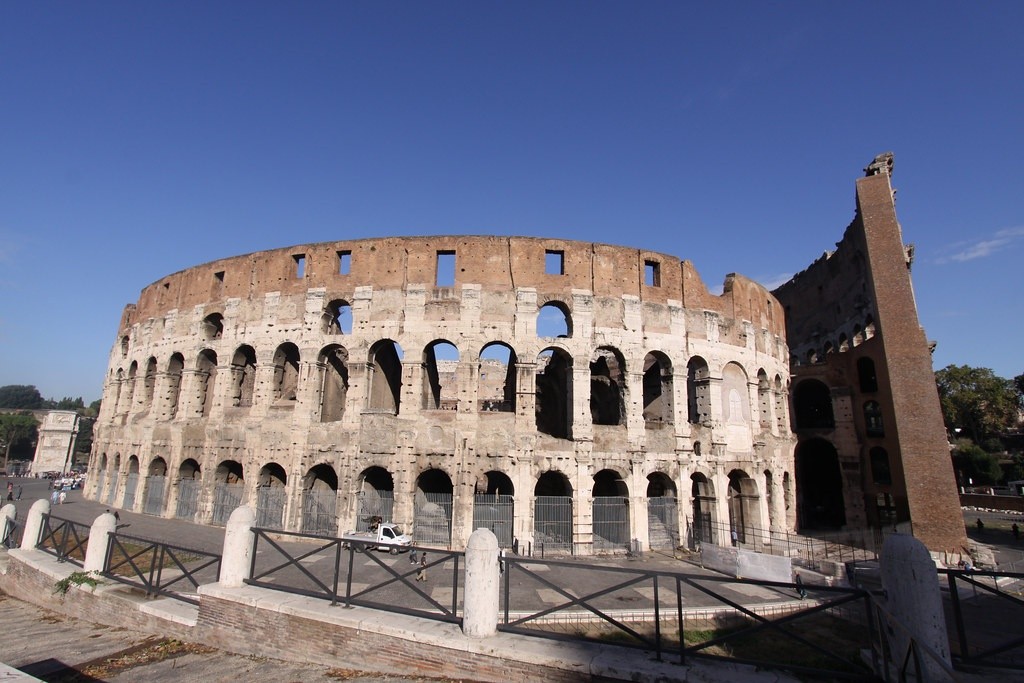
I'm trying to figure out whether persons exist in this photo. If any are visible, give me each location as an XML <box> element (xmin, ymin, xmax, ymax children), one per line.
<box><xmin>16</xmin><ymin>484</ymin><xmax>23</xmax><ymax>501</ymax></box>
<box><xmin>411</xmin><ymin>541</ymin><xmax>419</xmax><ymax>565</ymax></box>
<box><xmin>499</xmin><ymin>546</ymin><xmax>505</xmax><ymax>577</ymax></box>
<box><xmin>1012</xmin><ymin>524</ymin><xmax>1019</xmax><ymax>539</ymax></box>
<box><xmin>416</xmin><ymin>552</ymin><xmax>428</xmax><ymax>582</ymax></box>
<box><xmin>43</xmin><ymin>470</ymin><xmax>86</xmax><ymax>505</ymax></box>
<box><xmin>977</xmin><ymin>519</ymin><xmax>984</xmax><ymax>534</ymax></box>
<box><xmin>793</xmin><ymin>569</ymin><xmax>807</xmax><ymax>601</ymax></box>
<box><xmin>114</xmin><ymin>511</ymin><xmax>120</xmax><ymax>524</ymax></box>
<box><xmin>7</xmin><ymin>483</ymin><xmax>13</xmax><ymax>501</ymax></box>
<box><xmin>731</xmin><ymin>529</ymin><xmax>738</xmax><ymax>547</ymax></box>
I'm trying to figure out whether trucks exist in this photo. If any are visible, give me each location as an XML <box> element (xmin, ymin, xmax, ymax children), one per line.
<box><xmin>343</xmin><ymin>522</ymin><xmax>412</xmax><ymax>555</ymax></box>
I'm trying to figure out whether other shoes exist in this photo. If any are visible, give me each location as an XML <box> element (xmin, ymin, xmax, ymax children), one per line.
<box><xmin>411</xmin><ymin>563</ymin><xmax>414</xmax><ymax>564</ymax></box>
<box><xmin>415</xmin><ymin>562</ymin><xmax>419</xmax><ymax>565</ymax></box>
<box><xmin>423</xmin><ymin>580</ymin><xmax>428</xmax><ymax>582</ymax></box>
<box><xmin>16</xmin><ymin>499</ymin><xmax>21</xmax><ymax>500</ymax></box>
<box><xmin>800</xmin><ymin>595</ymin><xmax>807</xmax><ymax>599</ymax></box>
<box><xmin>416</xmin><ymin>579</ymin><xmax>419</xmax><ymax>582</ymax></box>
